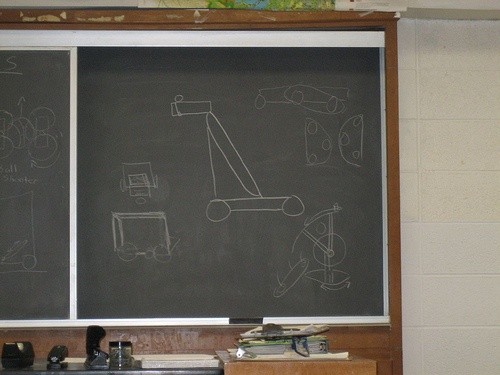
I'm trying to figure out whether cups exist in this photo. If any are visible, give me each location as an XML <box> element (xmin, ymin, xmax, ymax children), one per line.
<box><xmin>3</xmin><ymin>340</ymin><xmax>34</xmax><ymax>367</ymax></box>
<box><xmin>109</xmin><ymin>339</ymin><xmax>134</xmax><ymax>369</ymax></box>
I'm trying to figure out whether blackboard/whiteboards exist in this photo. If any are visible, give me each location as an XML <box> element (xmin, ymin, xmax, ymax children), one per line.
<box><xmin>70</xmin><ymin>46</ymin><xmax>392</xmax><ymax>326</ymax></box>
<box><xmin>0</xmin><ymin>45</ymin><xmax>77</xmax><ymax>326</ymax></box>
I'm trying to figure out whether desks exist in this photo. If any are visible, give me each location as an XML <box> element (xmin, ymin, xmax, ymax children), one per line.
<box><xmin>216</xmin><ymin>349</ymin><xmax>376</xmax><ymax>375</ymax></box>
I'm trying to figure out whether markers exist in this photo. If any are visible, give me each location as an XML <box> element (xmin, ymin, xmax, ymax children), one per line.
<box><xmin>230</xmin><ymin>316</ymin><xmax>263</xmax><ymax>324</ymax></box>
